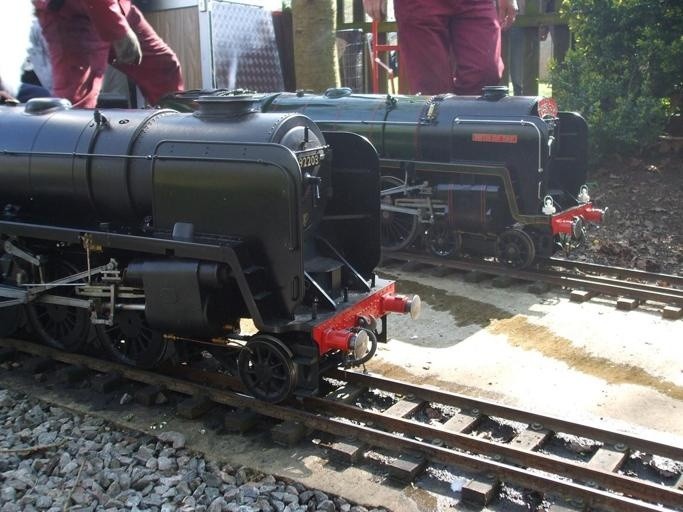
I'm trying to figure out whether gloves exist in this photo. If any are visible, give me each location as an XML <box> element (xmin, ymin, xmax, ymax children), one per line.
<box><xmin>115</xmin><ymin>29</ymin><xmax>143</xmax><ymax>66</ymax></box>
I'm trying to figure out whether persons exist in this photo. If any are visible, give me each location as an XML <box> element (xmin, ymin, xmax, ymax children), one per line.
<box><xmin>360</xmin><ymin>0</ymin><xmax>520</xmax><ymax>97</ymax></box>
<box><xmin>499</xmin><ymin>0</ymin><xmax>551</xmax><ymax>97</ymax></box>
<box><xmin>27</xmin><ymin>0</ymin><xmax>186</xmax><ymax>111</ymax></box>
